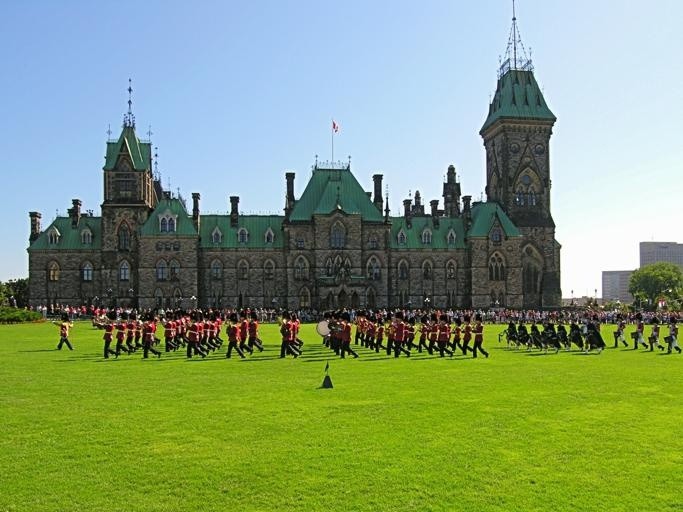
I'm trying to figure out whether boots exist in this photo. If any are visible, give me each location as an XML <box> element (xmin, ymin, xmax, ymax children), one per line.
<box><xmin>642</xmin><ymin>343</ymin><xmax>649</xmax><ymax>349</ymax></box>
<box><xmin>322</xmin><ymin>340</ymin><xmax>488</xmax><ymax>358</ymax></box>
<box><xmin>614</xmin><ymin>342</ymin><xmax>618</xmax><ymax>348</ymax></box>
<box><xmin>657</xmin><ymin>345</ymin><xmax>664</xmax><ymax>351</ymax></box>
<box><xmin>280</xmin><ymin>339</ymin><xmax>304</xmax><ymax>358</ymax></box>
<box><xmin>101</xmin><ymin>339</ymin><xmax>264</xmax><ymax>360</ymax></box>
<box><xmin>674</xmin><ymin>346</ymin><xmax>682</xmax><ymax>354</ymax></box>
<box><xmin>650</xmin><ymin>343</ymin><xmax>653</xmax><ymax>352</ymax></box>
<box><xmin>668</xmin><ymin>343</ymin><xmax>672</xmax><ymax>353</ymax></box>
<box><xmin>507</xmin><ymin>343</ymin><xmax>605</xmax><ymax>356</ymax></box>
<box><xmin>622</xmin><ymin>340</ymin><xmax>629</xmax><ymax>348</ymax></box>
<box><xmin>634</xmin><ymin>339</ymin><xmax>638</xmax><ymax>350</ymax></box>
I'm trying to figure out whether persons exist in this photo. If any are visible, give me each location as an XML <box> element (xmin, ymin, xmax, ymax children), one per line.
<box><xmin>648</xmin><ymin>317</ymin><xmax>664</xmax><ymax>351</ymax></box>
<box><xmin>506</xmin><ymin>320</ymin><xmax>606</xmax><ymax>355</ymax></box>
<box><xmin>280</xmin><ymin>310</ymin><xmax>303</xmax><ymax>358</ymax></box>
<box><xmin>25</xmin><ymin>301</ymin><xmax>94</xmax><ymax>322</ymax></box>
<box><xmin>322</xmin><ymin>311</ymin><xmax>359</xmax><ymax>359</ymax></box>
<box><xmin>631</xmin><ymin>313</ymin><xmax>648</xmax><ymax>350</ymax></box>
<box><xmin>95</xmin><ymin>311</ymin><xmax>161</xmax><ymax>358</ymax></box>
<box><xmin>613</xmin><ymin>317</ymin><xmax>628</xmax><ymax>348</ymax></box>
<box><xmin>94</xmin><ymin>306</ymin><xmax>683</xmax><ymax>323</ymax></box>
<box><xmin>226</xmin><ymin>312</ymin><xmax>264</xmax><ymax>359</ymax></box>
<box><xmin>353</xmin><ymin>311</ymin><xmax>488</xmax><ymax>359</ymax></box>
<box><xmin>666</xmin><ymin>317</ymin><xmax>682</xmax><ymax>354</ymax></box>
<box><xmin>159</xmin><ymin>310</ymin><xmax>223</xmax><ymax>358</ymax></box>
<box><xmin>53</xmin><ymin>316</ymin><xmax>76</xmax><ymax>350</ymax></box>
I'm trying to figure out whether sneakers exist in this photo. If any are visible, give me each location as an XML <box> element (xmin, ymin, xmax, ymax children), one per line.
<box><xmin>54</xmin><ymin>347</ymin><xmax>75</xmax><ymax>351</ymax></box>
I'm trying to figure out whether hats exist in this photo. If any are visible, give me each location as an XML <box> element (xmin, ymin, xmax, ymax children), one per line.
<box><xmin>617</xmin><ymin>314</ymin><xmax>622</xmax><ymax>320</ymax></box>
<box><xmin>653</xmin><ymin>317</ymin><xmax>661</xmax><ymax>323</ymax></box>
<box><xmin>354</xmin><ymin>310</ymin><xmax>482</xmax><ymax>323</ymax></box>
<box><xmin>283</xmin><ymin>310</ymin><xmax>300</xmax><ymax>321</ymax></box>
<box><xmin>324</xmin><ymin>310</ymin><xmax>351</xmax><ymax>323</ymax></box>
<box><xmin>636</xmin><ymin>313</ymin><xmax>642</xmax><ymax>321</ymax></box>
<box><xmin>670</xmin><ymin>317</ymin><xmax>676</xmax><ymax>322</ymax></box>
<box><xmin>60</xmin><ymin>312</ymin><xmax>69</xmax><ymax>320</ymax></box>
<box><xmin>105</xmin><ymin>309</ymin><xmax>257</xmax><ymax>324</ymax></box>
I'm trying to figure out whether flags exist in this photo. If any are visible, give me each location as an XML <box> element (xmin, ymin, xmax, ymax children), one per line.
<box><xmin>332</xmin><ymin>121</ymin><xmax>340</xmax><ymax>133</ymax></box>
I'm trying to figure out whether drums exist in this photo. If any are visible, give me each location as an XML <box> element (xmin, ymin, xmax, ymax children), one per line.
<box><xmin>648</xmin><ymin>337</ymin><xmax>657</xmax><ymax>343</ymax></box>
<box><xmin>631</xmin><ymin>332</ymin><xmax>639</xmax><ymax>338</ymax></box>
<box><xmin>316</xmin><ymin>321</ymin><xmax>331</xmax><ymax>336</ymax></box>
<box><xmin>614</xmin><ymin>332</ymin><xmax>621</xmax><ymax>337</ymax></box>
<box><xmin>664</xmin><ymin>336</ymin><xmax>673</xmax><ymax>342</ymax></box>
